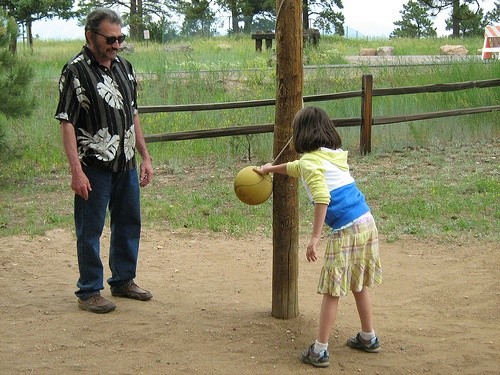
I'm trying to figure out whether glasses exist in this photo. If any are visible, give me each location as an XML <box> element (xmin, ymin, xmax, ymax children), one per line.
<box><xmin>93</xmin><ymin>29</ymin><xmax>126</xmax><ymax>46</ymax></box>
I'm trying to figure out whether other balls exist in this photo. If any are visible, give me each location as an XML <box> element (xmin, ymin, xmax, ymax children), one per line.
<box><xmin>233</xmin><ymin>165</ymin><xmax>273</xmax><ymax>205</ymax></box>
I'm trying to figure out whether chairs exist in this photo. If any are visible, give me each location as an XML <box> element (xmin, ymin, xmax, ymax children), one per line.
<box><xmin>486</xmin><ymin>36</ymin><xmax>500</xmax><ymax>62</ymax></box>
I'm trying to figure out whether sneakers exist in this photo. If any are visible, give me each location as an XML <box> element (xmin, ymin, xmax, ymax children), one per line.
<box><xmin>77</xmin><ymin>296</ymin><xmax>117</xmax><ymax>313</ymax></box>
<box><xmin>346</xmin><ymin>334</ymin><xmax>381</xmax><ymax>353</ymax></box>
<box><xmin>300</xmin><ymin>344</ymin><xmax>329</xmax><ymax>367</ymax></box>
<box><xmin>113</xmin><ymin>280</ymin><xmax>153</xmax><ymax>300</ymax></box>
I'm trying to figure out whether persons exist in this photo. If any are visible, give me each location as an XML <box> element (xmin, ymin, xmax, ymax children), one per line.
<box><xmin>252</xmin><ymin>107</ymin><xmax>382</xmax><ymax>366</ymax></box>
<box><xmin>55</xmin><ymin>8</ymin><xmax>152</xmax><ymax>313</ymax></box>
